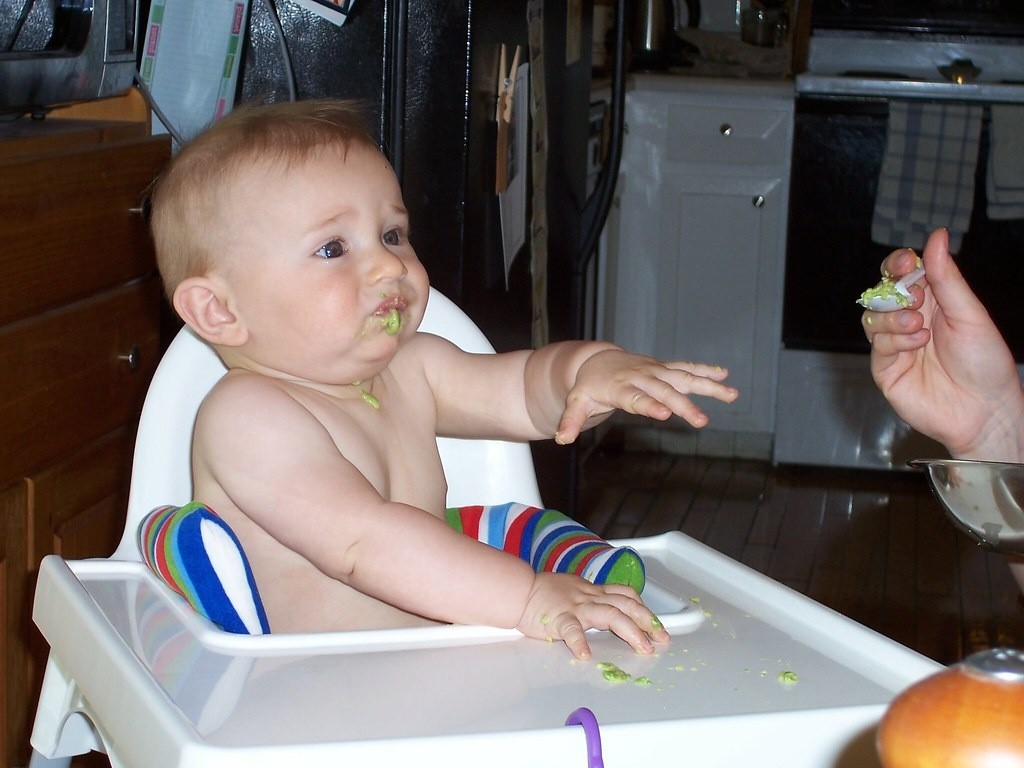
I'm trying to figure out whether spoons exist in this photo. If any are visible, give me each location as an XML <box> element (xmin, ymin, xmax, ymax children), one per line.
<box><xmin>857</xmin><ymin>258</ymin><xmax>926</xmax><ymax>312</ymax></box>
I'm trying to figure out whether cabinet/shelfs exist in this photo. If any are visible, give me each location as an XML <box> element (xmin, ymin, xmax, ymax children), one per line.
<box><xmin>0</xmin><ymin>116</ymin><xmax>174</xmax><ymax>768</ymax></box>
<box><xmin>581</xmin><ymin>71</ymin><xmax>1024</xmax><ymax>476</ymax></box>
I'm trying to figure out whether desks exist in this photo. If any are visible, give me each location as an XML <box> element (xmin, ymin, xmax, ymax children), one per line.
<box><xmin>32</xmin><ymin>531</ymin><xmax>954</xmax><ymax>768</ymax></box>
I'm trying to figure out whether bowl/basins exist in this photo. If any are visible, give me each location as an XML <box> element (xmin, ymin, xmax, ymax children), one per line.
<box><xmin>906</xmin><ymin>458</ymin><xmax>1024</xmax><ymax>557</ymax></box>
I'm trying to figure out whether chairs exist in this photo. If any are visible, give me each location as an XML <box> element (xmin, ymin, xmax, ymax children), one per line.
<box><xmin>23</xmin><ymin>285</ymin><xmax>548</xmax><ymax>768</ymax></box>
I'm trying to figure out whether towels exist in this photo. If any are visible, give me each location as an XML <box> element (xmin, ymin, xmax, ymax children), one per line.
<box><xmin>984</xmin><ymin>102</ymin><xmax>1024</xmax><ymax>222</ymax></box>
<box><xmin>870</xmin><ymin>99</ymin><xmax>984</xmax><ymax>258</ymax></box>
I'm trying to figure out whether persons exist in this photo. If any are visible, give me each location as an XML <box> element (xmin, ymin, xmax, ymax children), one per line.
<box><xmin>861</xmin><ymin>227</ymin><xmax>1024</xmax><ymax>461</ymax></box>
<box><xmin>150</xmin><ymin>103</ymin><xmax>737</xmax><ymax>659</ymax></box>
